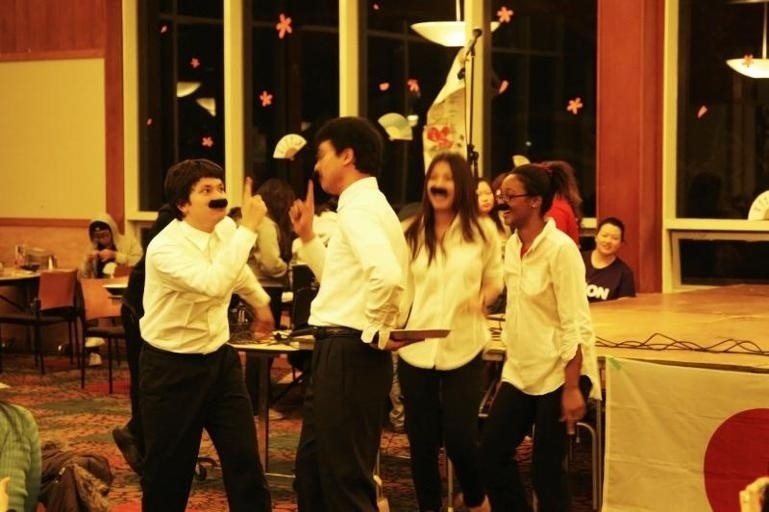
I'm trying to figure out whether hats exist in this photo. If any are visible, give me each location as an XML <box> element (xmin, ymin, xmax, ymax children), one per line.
<box><xmin>91</xmin><ymin>221</ymin><xmax>108</xmax><ymax>231</ymax></box>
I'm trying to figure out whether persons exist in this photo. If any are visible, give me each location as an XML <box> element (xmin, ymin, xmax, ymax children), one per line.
<box><xmin>112</xmin><ymin>157</ymin><xmax>298</xmax><ymax>511</ymax></box>
<box><xmin>289</xmin><ymin>114</ymin><xmax>607</xmax><ymax>510</ymax></box>
<box><xmin>579</xmin><ymin>216</ymin><xmax>635</xmax><ymax>305</ymax></box>
<box><xmin>76</xmin><ymin>214</ymin><xmax>145</xmax><ymax>368</ymax></box>
<box><xmin>0</xmin><ymin>382</ymin><xmax>42</xmax><ymax>512</ymax></box>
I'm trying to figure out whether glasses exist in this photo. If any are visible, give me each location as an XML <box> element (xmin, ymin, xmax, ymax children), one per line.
<box><xmin>495</xmin><ymin>190</ymin><xmax>530</xmax><ymax>202</ymax></box>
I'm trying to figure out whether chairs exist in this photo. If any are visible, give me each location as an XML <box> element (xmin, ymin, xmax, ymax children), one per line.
<box><xmin>79</xmin><ymin>279</ymin><xmax>126</xmax><ymax>394</ymax></box>
<box><xmin>3</xmin><ymin>267</ymin><xmax>79</xmax><ymax>375</ymax></box>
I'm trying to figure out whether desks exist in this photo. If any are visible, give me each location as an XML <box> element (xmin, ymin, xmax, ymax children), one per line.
<box><xmin>3</xmin><ymin>268</ymin><xmax>40</xmax><ymax>288</ymax></box>
<box><xmin>101</xmin><ymin>275</ymin><xmax>129</xmax><ymax>290</ymax></box>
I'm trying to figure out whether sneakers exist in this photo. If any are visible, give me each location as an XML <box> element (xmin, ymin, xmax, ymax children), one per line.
<box><xmin>84</xmin><ymin>337</ymin><xmax>106</xmax><ymax>348</ymax></box>
<box><xmin>89</xmin><ymin>352</ymin><xmax>103</xmax><ymax>367</ymax></box>
<box><xmin>111</xmin><ymin>425</ymin><xmax>145</xmax><ymax>475</ymax></box>
<box><xmin>192</xmin><ymin>463</ymin><xmax>207</xmax><ymax>485</ymax></box>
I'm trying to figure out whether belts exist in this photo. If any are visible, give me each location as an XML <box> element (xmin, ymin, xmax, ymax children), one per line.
<box><xmin>313</xmin><ymin>326</ymin><xmax>360</xmax><ymax>341</ymax></box>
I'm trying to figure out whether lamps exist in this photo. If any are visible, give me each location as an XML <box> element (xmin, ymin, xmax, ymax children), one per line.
<box><xmin>411</xmin><ymin>0</ymin><xmax>501</xmax><ymax>49</ymax></box>
<box><xmin>726</xmin><ymin>1</ymin><xmax>769</xmax><ymax>80</ymax></box>
